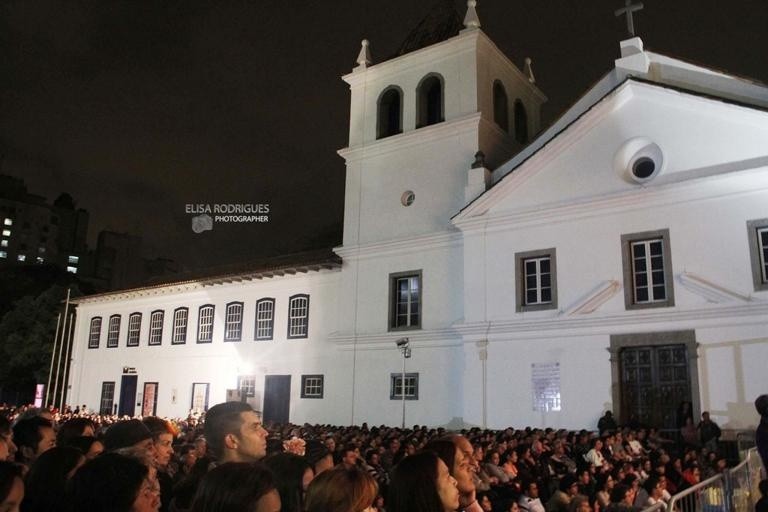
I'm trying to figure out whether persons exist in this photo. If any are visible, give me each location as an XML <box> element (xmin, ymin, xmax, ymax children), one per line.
<box><xmin>0</xmin><ymin>395</ymin><xmax>768</xmax><ymax>511</ymax></box>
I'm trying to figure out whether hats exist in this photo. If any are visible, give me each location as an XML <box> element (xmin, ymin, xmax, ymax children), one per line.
<box><xmin>103</xmin><ymin>418</ymin><xmax>152</xmax><ymax>451</ymax></box>
